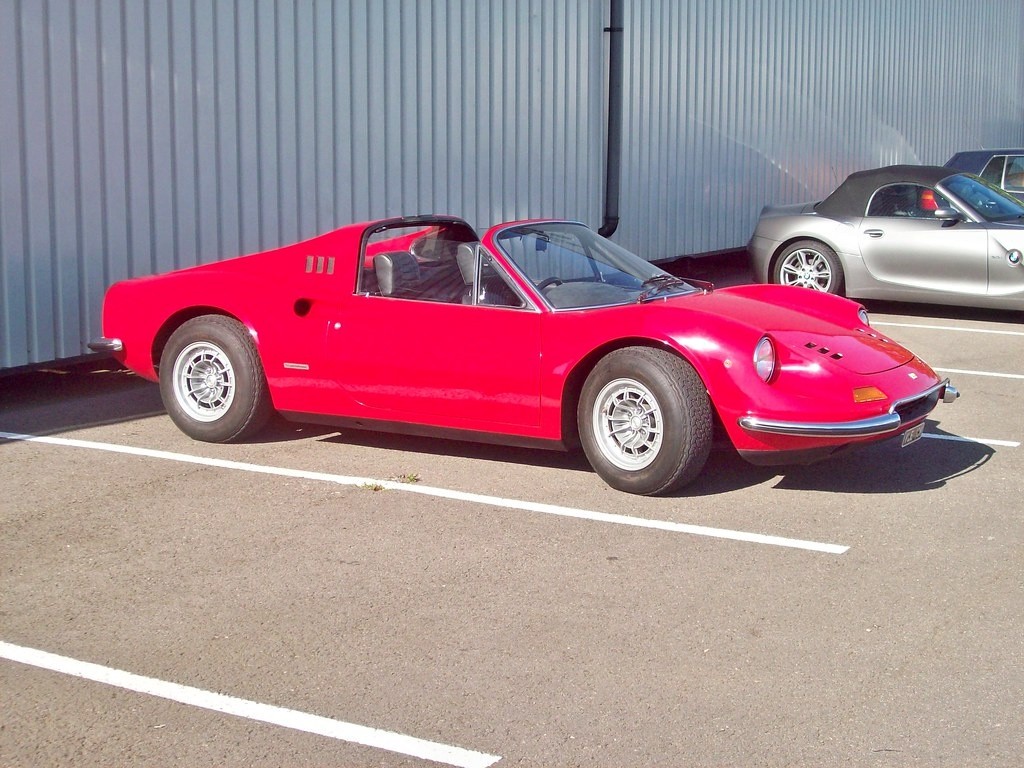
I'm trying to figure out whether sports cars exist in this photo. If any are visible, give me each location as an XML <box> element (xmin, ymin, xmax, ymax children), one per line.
<box><xmin>743</xmin><ymin>162</ymin><xmax>1024</xmax><ymax>320</ymax></box>
<box><xmin>84</xmin><ymin>213</ymin><xmax>963</xmax><ymax>498</ymax></box>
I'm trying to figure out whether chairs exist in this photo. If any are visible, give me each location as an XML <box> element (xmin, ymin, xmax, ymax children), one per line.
<box><xmin>374</xmin><ymin>251</ymin><xmax>420</xmax><ymax>297</ymax></box>
<box><xmin>456</xmin><ymin>242</ymin><xmax>519</xmax><ymax>304</ymax></box>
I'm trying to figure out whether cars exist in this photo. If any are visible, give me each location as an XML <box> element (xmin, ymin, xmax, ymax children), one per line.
<box><xmin>919</xmin><ymin>148</ymin><xmax>1024</xmax><ymax>226</ymax></box>
